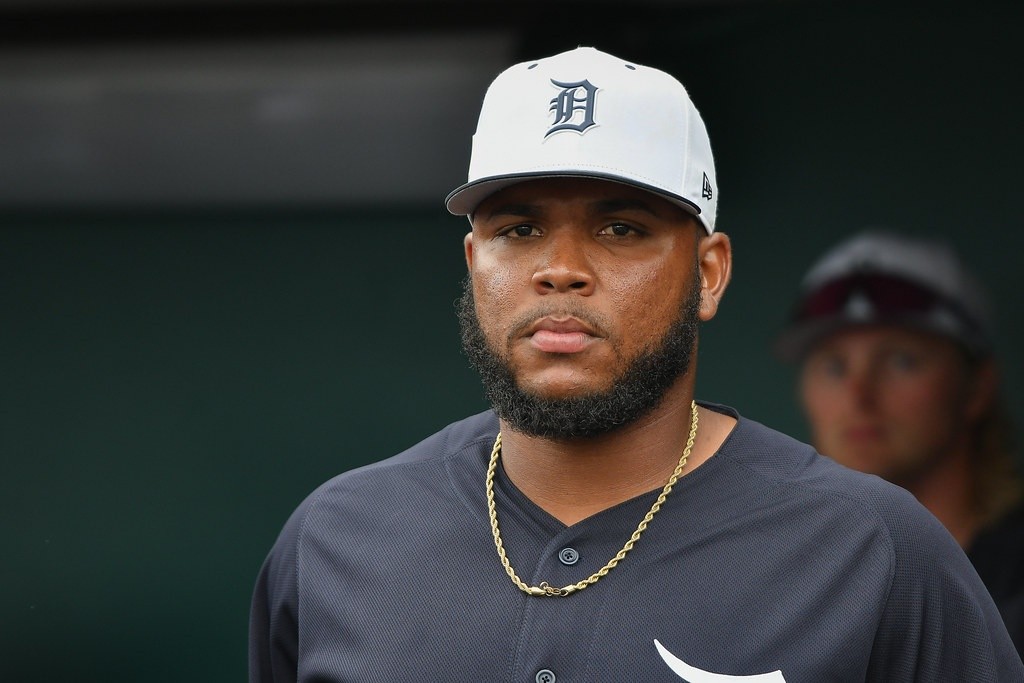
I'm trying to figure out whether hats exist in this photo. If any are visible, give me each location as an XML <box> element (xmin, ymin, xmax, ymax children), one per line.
<box><xmin>445</xmin><ymin>45</ymin><xmax>718</xmax><ymax>236</ymax></box>
<box><xmin>773</xmin><ymin>233</ymin><xmax>996</xmax><ymax>357</ymax></box>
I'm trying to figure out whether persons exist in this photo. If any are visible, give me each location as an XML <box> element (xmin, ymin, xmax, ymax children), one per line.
<box><xmin>780</xmin><ymin>235</ymin><xmax>1023</xmax><ymax>655</ymax></box>
<box><xmin>247</xmin><ymin>43</ymin><xmax>1023</xmax><ymax>683</ymax></box>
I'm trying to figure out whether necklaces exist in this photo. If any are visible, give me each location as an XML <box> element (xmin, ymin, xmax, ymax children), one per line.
<box><xmin>485</xmin><ymin>398</ymin><xmax>699</xmax><ymax>597</ymax></box>
